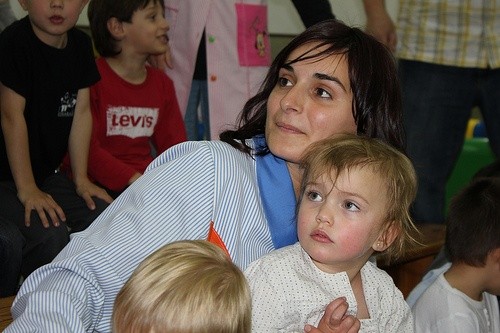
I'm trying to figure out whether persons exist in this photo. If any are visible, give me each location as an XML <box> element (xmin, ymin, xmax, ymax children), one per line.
<box><xmin>0</xmin><ymin>0</ymin><xmax>500</xmax><ymax>333</ymax></box>
